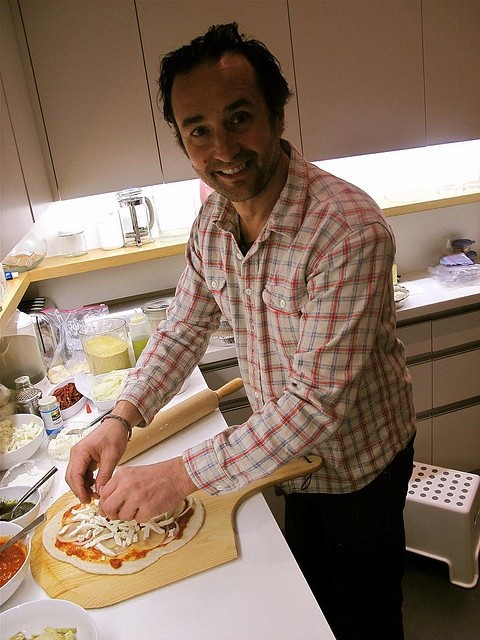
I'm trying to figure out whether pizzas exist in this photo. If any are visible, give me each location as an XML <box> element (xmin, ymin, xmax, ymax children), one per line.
<box><xmin>40</xmin><ymin>495</ymin><xmax>205</xmax><ymax>576</ymax></box>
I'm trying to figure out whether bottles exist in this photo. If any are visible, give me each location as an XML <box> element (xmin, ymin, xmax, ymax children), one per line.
<box><xmin>129</xmin><ymin>309</ymin><xmax>152</xmax><ymax>363</ymax></box>
<box><xmin>141</xmin><ymin>296</ymin><xmax>179</xmax><ymax>342</ymax></box>
<box><xmin>12</xmin><ymin>376</ymin><xmax>40</xmax><ymax>417</ymax></box>
<box><xmin>0</xmin><ymin>262</ymin><xmax>7</xmax><ymax>312</ymax></box>
<box><xmin>35</xmin><ymin>395</ymin><xmax>68</xmax><ymax>437</ymax></box>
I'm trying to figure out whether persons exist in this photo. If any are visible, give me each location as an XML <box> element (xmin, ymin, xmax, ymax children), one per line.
<box><xmin>62</xmin><ymin>18</ymin><xmax>418</xmax><ymax>640</ymax></box>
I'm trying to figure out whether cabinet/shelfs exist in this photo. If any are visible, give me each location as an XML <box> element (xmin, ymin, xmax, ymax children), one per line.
<box><xmin>432</xmin><ymin>305</ymin><xmax>480</xmax><ymax>474</ymax></box>
<box><xmin>133</xmin><ymin>0</ymin><xmax>303</xmax><ymax>184</ymax></box>
<box><xmin>1</xmin><ymin>1</ymin><xmax>59</xmax><ymax>223</ymax></box>
<box><xmin>286</xmin><ymin>1</ymin><xmax>422</xmax><ymax>163</ymax></box>
<box><xmin>19</xmin><ymin>1</ymin><xmax>164</xmax><ymax>201</ymax></box>
<box><xmin>421</xmin><ymin>1</ymin><xmax>480</xmax><ymax>147</ymax></box>
<box><xmin>0</xmin><ymin>90</ymin><xmax>35</xmax><ymax>263</ymax></box>
<box><xmin>202</xmin><ymin>363</ymin><xmax>285</xmax><ymax>529</ymax></box>
<box><xmin>394</xmin><ymin>314</ymin><xmax>432</xmax><ymax>466</ymax></box>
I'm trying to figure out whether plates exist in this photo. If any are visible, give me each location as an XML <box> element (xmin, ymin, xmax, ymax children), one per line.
<box><xmin>0</xmin><ymin>598</ymin><xmax>101</xmax><ymax>640</ymax></box>
<box><xmin>46</xmin><ymin>378</ymin><xmax>88</xmax><ymax>421</ymax></box>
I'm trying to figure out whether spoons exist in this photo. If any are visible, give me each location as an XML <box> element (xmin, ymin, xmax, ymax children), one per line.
<box><xmin>65</xmin><ymin>409</ymin><xmax>113</xmax><ymax>438</ymax></box>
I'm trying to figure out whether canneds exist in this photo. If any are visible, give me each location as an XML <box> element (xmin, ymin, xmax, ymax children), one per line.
<box><xmin>141</xmin><ymin>297</ymin><xmax>176</xmax><ymax>334</ymax></box>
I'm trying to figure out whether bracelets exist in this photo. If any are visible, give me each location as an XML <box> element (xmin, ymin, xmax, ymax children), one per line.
<box><xmin>100</xmin><ymin>414</ymin><xmax>132</xmax><ymax>443</ymax></box>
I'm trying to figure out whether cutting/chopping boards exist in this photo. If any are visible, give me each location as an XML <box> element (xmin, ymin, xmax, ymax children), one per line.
<box><xmin>30</xmin><ymin>453</ymin><xmax>322</xmax><ymax>610</ymax></box>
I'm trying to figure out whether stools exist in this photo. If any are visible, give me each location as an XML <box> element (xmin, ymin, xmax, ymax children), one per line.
<box><xmin>402</xmin><ymin>460</ymin><xmax>480</xmax><ymax>591</ymax></box>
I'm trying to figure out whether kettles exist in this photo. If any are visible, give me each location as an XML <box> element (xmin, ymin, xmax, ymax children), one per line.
<box><xmin>0</xmin><ymin>308</ymin><xmax>55</xmax><ymax>401</ymax></box>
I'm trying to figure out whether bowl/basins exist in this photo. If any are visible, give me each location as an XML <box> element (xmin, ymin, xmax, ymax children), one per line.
<box><xmin>0</xmin><ymin>486</ymin><xmax>43</xmax><ymax>531</ymax></box>
<box><xmin>465</xmin><ymin>251</ymin><xmax>477</xmax><ymax>262</ymax></box>
<box><xmin>393</xmin><ymin>283</ymin><xmax>410</xmax><ymax>311</ymax></box>
<box><xmin>73</xmin><ymin>376</ymin><xmax>130</xmax><ymax>408</ymax></box>
<box><xmin>452</xmin><ymin>240</ymin><xmax>474</xmax><ymax>254</ymax></box>
<box><xmin>0</xmin><ymin>412</ymin><xmax>46</xmax><ymax>471</ymax></box>
<box><xmin>0</xmin><ymin>523</ymin><xmax>33</xmax><ymax>611</ymax></box>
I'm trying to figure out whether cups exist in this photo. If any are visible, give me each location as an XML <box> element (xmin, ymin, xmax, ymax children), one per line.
<box><xmin>116</xmin><ymin>185</ymin><xmax>157</xmax><ymax>247</ymax></box>
<box><xmin>55</xmin><ymin>224</ymin><xmax>88</xmax><ymax>258</ymax></box>
<box><xmin>98</xmin><ymin>210</ymin><xmax>125</xmax><ymax>250</ymax></box>
<box><xmin>76</xmin><ymin>315</ymin><xmax>136</xmax><ymax>375</ymax></box>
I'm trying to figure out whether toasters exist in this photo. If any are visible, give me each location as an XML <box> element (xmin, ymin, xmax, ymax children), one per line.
<box><xmin>17</xmin><ymin>296</ymin><xmax>71</xmax><ymax>367</ymax></box>
